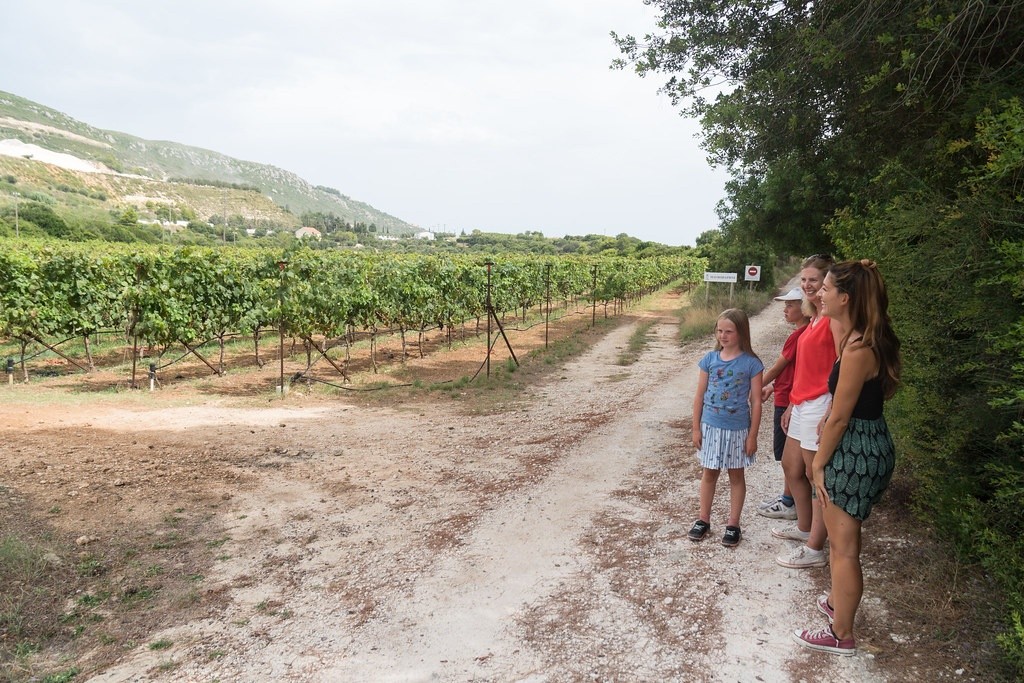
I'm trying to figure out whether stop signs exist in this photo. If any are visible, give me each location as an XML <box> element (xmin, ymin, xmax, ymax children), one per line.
<box><xmin>745</xmin><ymin>265</ymin><xmax>761</xmax><ymax>281</ymax></box>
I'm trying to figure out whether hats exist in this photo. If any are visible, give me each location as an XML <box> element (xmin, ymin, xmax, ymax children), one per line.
<box><xmin>774</xmin><ymin>288</ymin><xmax>804</xmax><ymax>301</ymax></box>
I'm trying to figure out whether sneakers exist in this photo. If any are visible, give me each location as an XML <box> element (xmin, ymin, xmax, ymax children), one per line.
<box><xmin>756</xmin><ymin>497</ymin><xmax>797</xmax><ymax>521</ymax></box>
<box><xmin>721</xmin><ymin>525</ymin><xmax>742</xmax><ymax>546</ymax></box>
<box><xmin>776</xmin><ymin>543</ymin><xmax>827</xmax><ymax>568</ymax></box>
<box><xmin>688</xmin><ymin>520</ymin><xmax>710</xmax><ymax>540</ymax></box>
<box><xmin>792</xmin><ymin>623</ymin><xmax>856</xmax><ymax>657</ymax></box>
<box><xmin>771</xmin><ymin>526</ymin><xmax>809</xmax><ymax>542</ymax></box>
<box><xmin>816</xmin><ymin>594</ymin><xmax>835</xmax><ymax>624</ymax></box>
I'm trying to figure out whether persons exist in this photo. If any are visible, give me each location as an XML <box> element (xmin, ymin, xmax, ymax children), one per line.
<box><xmin>790</xmin><ymin>260</ymin><xmax>905</xmax><ymax>656</ymax></box>
<box><xmin>770</xmin><ymin>254</ymin><xmax>845</xmax><ymax>570</ymax></box>
<box><xmin>686</xmin><ymin>307</ymin><xmax>764</xmax><ymax>546</ymax></box>
<box><xmin>750</xmin><ymin>287</ymin><xmax>798</xmax><ymax>521</ymax></box>
